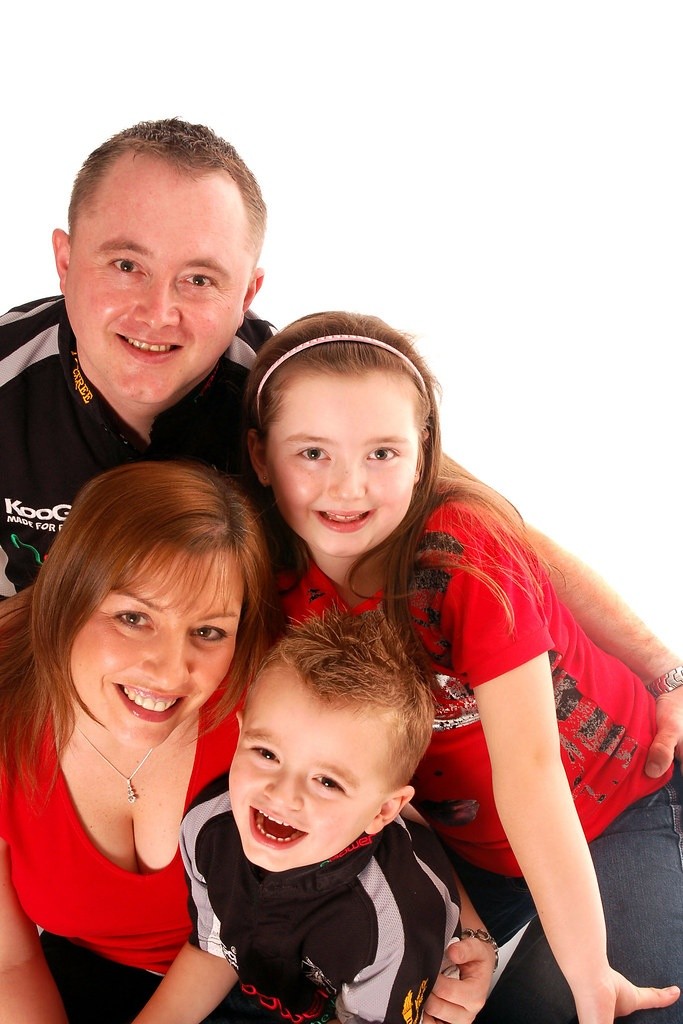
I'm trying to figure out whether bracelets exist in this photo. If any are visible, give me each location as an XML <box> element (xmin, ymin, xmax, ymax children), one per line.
<box><xmin>459</xmin><ymin>927</ymin><xmax>499</xmax><ymax>973</ymax></box>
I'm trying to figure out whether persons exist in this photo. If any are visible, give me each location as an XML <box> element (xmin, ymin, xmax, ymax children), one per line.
<box><xmin>0</xmin><ymin>116</ymin><xmax>683</xmax><ymax>1024</ymax></box>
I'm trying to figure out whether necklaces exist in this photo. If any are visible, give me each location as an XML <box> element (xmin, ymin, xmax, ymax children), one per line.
<box><xmin>73</xmin><ymin>721</ymin><xmax>154</xmax><ymax>804</ymax></box>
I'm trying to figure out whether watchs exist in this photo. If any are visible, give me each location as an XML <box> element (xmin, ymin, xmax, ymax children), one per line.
<box><xmin>645</xmin><ymin>665</ymin><xmax>683</xmax><ymax>698</ymax></box>
